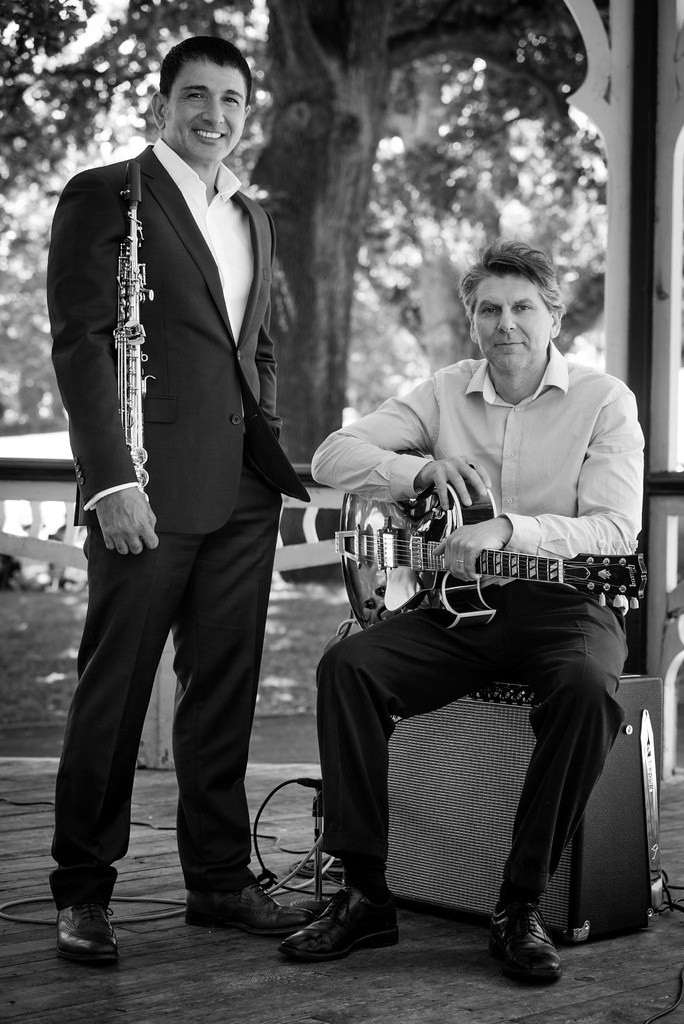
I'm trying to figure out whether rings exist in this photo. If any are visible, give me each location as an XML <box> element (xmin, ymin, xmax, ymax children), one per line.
<box><xmin>455</xmin><ymin>560</ymin><xmax>464</xmax><ymax>563</ymax></box>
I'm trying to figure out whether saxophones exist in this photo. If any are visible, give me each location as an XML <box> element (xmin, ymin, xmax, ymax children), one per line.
<box><xmin>111</xmin><ymin>159</ymin><xmax>155</xmax><ymax>500</ymax></box>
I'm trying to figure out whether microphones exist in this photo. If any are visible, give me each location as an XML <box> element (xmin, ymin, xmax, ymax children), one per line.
<box><xmin>297</xmin><ymin>778</ymin><xmax>324</xmax><ymax>792</ymax></box>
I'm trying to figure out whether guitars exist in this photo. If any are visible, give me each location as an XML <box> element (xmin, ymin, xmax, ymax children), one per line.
<box><xmin>334</xmin><ymin>448</ymin><xmax>649</xmax><ymax>638</ymax></box>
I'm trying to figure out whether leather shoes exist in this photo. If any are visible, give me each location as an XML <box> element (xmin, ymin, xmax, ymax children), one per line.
<box><xmin>279</xmin><ymin>885</ymin><xmax>398</xmax><ymax>959</ymax></box>
<box><xmin>184</xmin><ymin>885</ymin><xmax>315</xmax><ymax>937</ymax></box>
<box><xmin>56</xmin><ymin>903</ymin><xmax>121</xmax><ymax>960</ymax></box>
<box><xmin>489</xmin><ymin>902</ymin><xmax>561</xmax><ymax>975</ymax></box>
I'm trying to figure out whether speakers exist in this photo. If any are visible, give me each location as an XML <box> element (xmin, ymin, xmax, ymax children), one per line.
<box><xmin>388</xmin><ymin>673</ymin><xmax>662</xmax><ymax>939</ymax></box>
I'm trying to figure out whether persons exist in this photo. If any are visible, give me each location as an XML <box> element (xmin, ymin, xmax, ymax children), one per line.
<box><xmin>46</xmin><ymin>35</ymin><xmax>315</xmax><ymax>961</ymax></box>
<box><xmin>277</xmin><ymin>243</ymin><xmax>647</xmax><ymax>985</ymax></box>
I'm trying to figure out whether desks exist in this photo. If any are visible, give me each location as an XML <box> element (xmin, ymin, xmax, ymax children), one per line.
<box><xmin>0</xmin><ymin>431</ymin><xmax>347</xmax><ymax>767</ymax></box>
